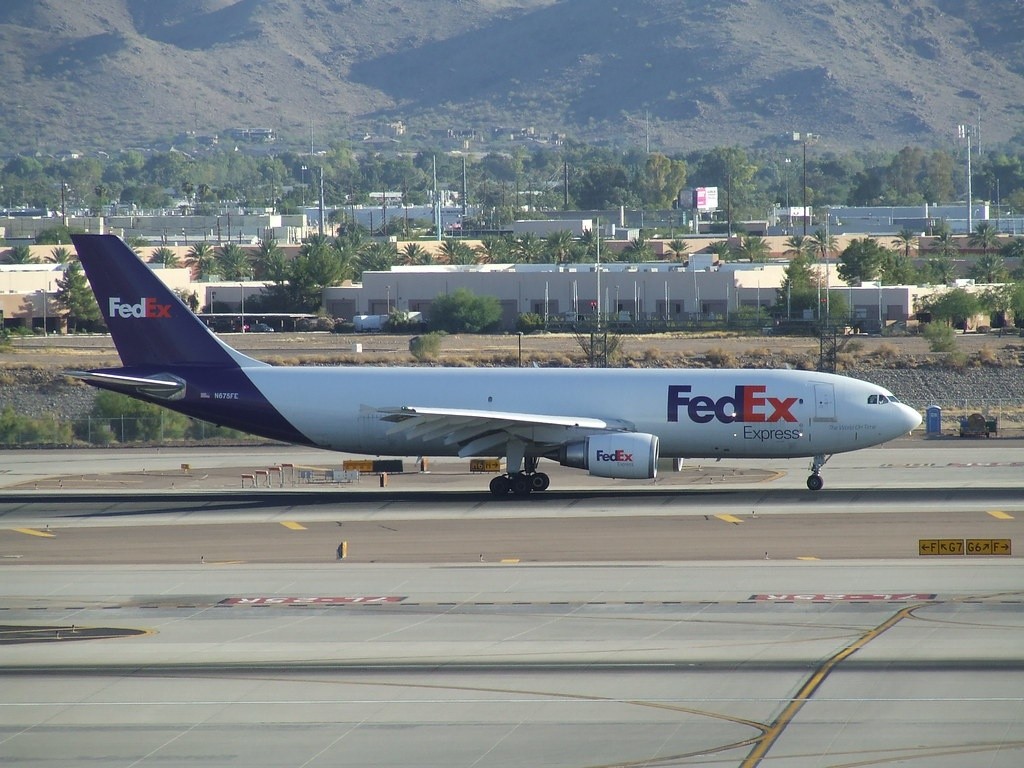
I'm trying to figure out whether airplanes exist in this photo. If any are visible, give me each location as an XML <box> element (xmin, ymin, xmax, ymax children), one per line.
<box><xmin>59</xmin><ymin>234</ymin><xmax>925</xmax><ymax>498</ymax></box>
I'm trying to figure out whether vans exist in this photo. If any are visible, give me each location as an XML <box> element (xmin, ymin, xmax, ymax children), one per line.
<box><xmin>213</xmin><ymin>317</ymin><xmax>250</xmax><ymax>333</ymax></box>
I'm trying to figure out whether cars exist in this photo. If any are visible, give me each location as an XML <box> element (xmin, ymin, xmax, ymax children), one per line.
<box><xmin>249</xmin><ymin>323</ymin><xmax>274</xmax><ymax>332</ymax></box>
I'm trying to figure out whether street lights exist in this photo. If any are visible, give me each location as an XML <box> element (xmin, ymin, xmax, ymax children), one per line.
<box><xmin>301</xmin><ymin>165</ymin><xmax>308</xmax><ymax>213</ymax></box>
<box><xmin>791</xmin><ymin>132</ymin><xmax>815</xmax><ymax>234</ymax></box>
<box><xmin>958</xmin><ymin>122</ymin><xmax>980</xmax><ymax>235</ymax></box>
